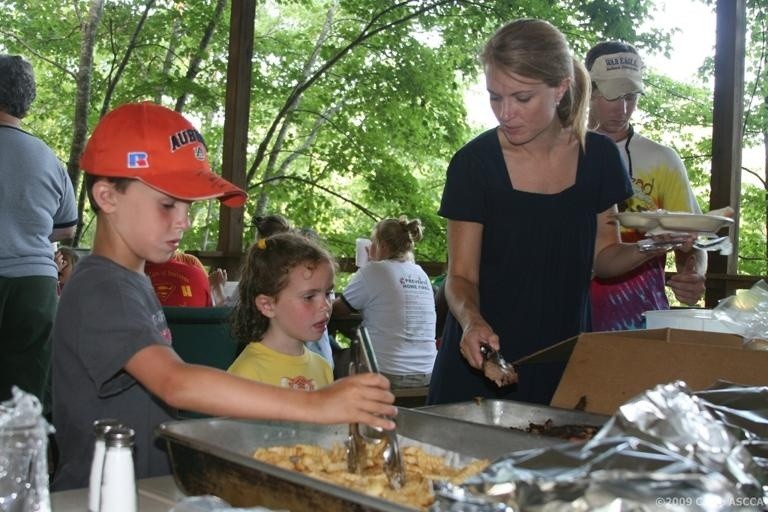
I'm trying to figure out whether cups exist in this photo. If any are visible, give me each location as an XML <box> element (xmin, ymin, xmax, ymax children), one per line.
<box><xmin>354</xmin><ymin>237</ymin><xmax>372</xmax><ymax>267</ymax></box>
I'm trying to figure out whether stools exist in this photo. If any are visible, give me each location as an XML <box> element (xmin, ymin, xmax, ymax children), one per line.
<box><xmin>390</xmin><ymin>385</ymin><xmax>430</xmax><ymax>403</ymax></box>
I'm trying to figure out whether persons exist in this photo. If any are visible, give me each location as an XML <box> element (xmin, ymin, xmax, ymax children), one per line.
<box><xmin>583</xmin><ymin>40</ymin><xmax>710</xmax><ymax>330</ymax></box>
<box><xmin>54</xmin><ymin>246</ymin><xmax>81</xmax><ymax>297</ymax></box>
<box><xmin>1</xmin><ymin>54</ymin><xmax>80</xmax><ymax>402</ymax></box>
<box><xmin>172</xmin><ymin>252</ymin><xmax>209</xmax><ymax>279</ymax></box>
<box><xmin>434</xmin><ymin>254</ymin><xmax>450</xmax><ymax>351</ymax></box>
<box><xmin>225</xmin><ymin>231</ymin><xmax>336</xmax><ymax>392</ymax></box>
<box><xmin>208</xmin><ymin>214</ymin><xmax>294</xmax><ymax>307</ymax></box>
<box><xmin>49</xmin><ymin>103</ymin><xmax>399</xmax><ymax>492</ymax></box>
<box><xmin>426</xmin><ymin>19</ymin><xmax>699</xmax><ymax>405</ymax></box>
<box><xmin>145</xmin><ymin>260</ymin><xmax>213</xmax><ymax>308</ymax></box>
<box><xmin>332</xmin><ymin>218</ymin><xmax>439</xmax><ymax>389</ymax></box>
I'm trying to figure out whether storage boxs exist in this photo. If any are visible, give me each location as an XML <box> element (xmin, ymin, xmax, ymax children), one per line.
<box><xmin>511</xmin><ymin>326</ymin><xmax>768</xmax><ymax>416</ymax></box>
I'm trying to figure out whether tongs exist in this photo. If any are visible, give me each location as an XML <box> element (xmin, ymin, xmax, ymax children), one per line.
<box><xmin>478</xmin><ymin>345</ymin><xmax>517</xmax><ymax>388</ymax></box>
<box><xmin>345</xmin><ymin>326</ymin><xmax>405</xmax><ymax>491</ymax></box>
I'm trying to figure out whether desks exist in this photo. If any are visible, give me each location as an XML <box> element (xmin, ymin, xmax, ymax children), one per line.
<box><xmin>50</xmin><ymin>474</ymin><xmax>196</xmax><ymax>512</ymax></box>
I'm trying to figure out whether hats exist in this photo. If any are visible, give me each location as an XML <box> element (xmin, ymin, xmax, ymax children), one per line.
<box><xmin>79</xmin><ymin>101</ymin><xmax>248</xmax><ymax>209</ymax></box>
<box><xmin>585</xmin><ymin>41</ymin><xmax>648</xmax><ymax>102</ymax></box>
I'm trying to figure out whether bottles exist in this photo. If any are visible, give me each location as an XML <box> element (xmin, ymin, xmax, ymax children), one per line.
<box><xmin>86</xmin><ymin>418</ymin><xmax>139</xmax><ymax>512</ymax></box>
<box><xmin>0</xmin><ymin>408</ymin><xmax>50</xmax><ymax>510</ymax></box>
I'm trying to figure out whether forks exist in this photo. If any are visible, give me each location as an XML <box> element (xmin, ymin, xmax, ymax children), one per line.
<box><xmin>640</xmin><ymin>236</ymin><xmax>726</xmax><ymax>250</ymax></box>
